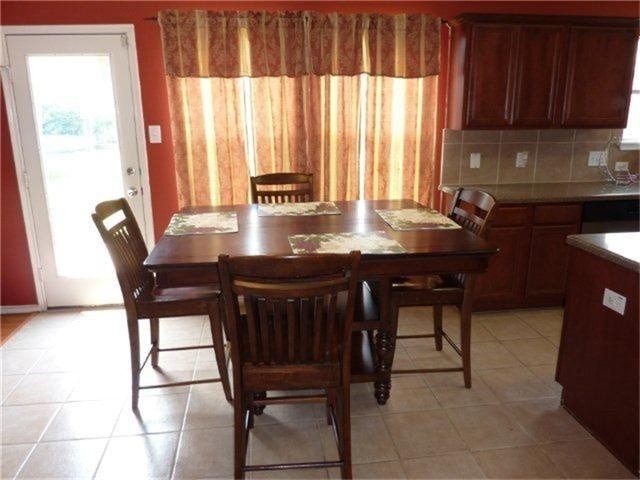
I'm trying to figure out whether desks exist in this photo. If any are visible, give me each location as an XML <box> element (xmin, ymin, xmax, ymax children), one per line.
<box><xmin>144</xmin><ymin>199</ymin><xmax>499</xmax><ymax>414</ymax></box>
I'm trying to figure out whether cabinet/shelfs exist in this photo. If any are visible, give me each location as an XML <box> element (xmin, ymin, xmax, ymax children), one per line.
<box><xmin>447</xmin><ymin>12</ymin><xmax>570</xmax><ymax>130</ymax></box>
<box><xmin>443</xmin><ymin>194</ymin><xmax>583</xmax><ymax>312</ymax></box>
<box><xmin>560</xmin><ymin>14</ymin><xmax>639</xmax><ymax>130</ymax></box>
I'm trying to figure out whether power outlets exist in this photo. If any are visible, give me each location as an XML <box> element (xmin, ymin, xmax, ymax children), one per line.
<box><xmin>588</xmin><ymin>151</ymin><xmax>608</xmax><ymax>166</ymax></box>
<box><xmin>615</xmin><ymin>161</ymin><xmax>630</xmax><ymax>172</ymax></box>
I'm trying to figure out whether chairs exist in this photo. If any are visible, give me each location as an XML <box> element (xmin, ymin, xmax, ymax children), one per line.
<box><xmin>369</xmin><ymin>187</ymin><xmax>499</xmax><ymax>387</ymax></box>
<box><xmin>218</xmin><ymin>250</ymin><xmax>361</xmax><ymax>480</ymax></box>
<box><xmin>92</xmin><ymin>198</ymin><xmax>232</xmax><ymax>410</ymax></box>
<box><xmin>251</xmin><ymin>173</ymin><xmax>314</xmax><ymax>204</ymax></box>
